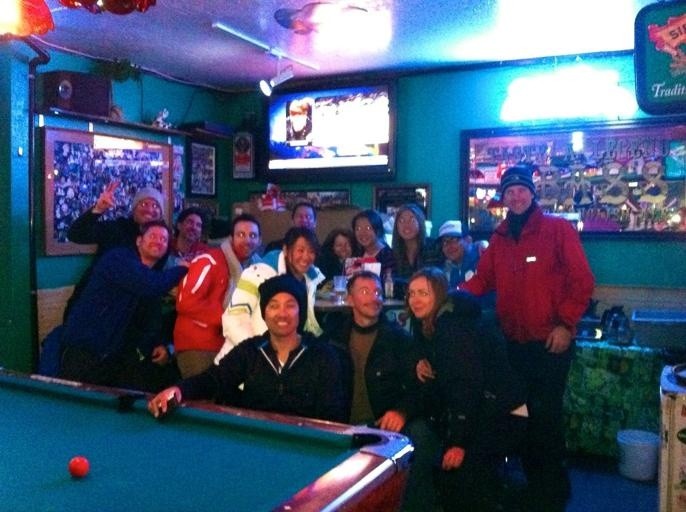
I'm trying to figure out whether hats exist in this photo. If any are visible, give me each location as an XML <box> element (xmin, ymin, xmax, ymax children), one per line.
<box><xmin>500</xmin><ymin>163</ymin><xmax>536</xmax><ymax>197</ymax></box>
<box><xmin>436</xmin><ymin>220</ymin><xmax>462</xmax><ymax>239</ymax></box>
<box><xmin>258</xmin><ymin>273</ymin><xmax>310</xmax><ymax>323</ymax></box>
<box><xmin>129</xmin><ymin>186</ymin><xmax>164</xmax><ymax>218</ymax></box>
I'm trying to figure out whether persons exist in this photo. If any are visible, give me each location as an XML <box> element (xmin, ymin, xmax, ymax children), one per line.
<box><xmin>385</xmin><ymin>201</ymin><xmax>434</xmax><ymax>287</ymax></box>
<box><xmin>456</xmin><ymin>166</ymin><xmax>596</xmax><ymax>512</ymax></box>
<box><xmin>408</xmin><ymin>269</ymin><xmax>539</xmax><ymax>510</ymax></box>
<box><xmin>351</xmin><ymin>209</ymin><xmax>394</xmax><ymax>282</ymax></box>
<box><xmin>263</xmin><ymin>202</ymin><xmax>320</xmax><ymax>255</ymax></box>
<box><xmin>212</xmin><ymin>226</ymin><xmax>326</xmax><ymax>366</ymax></box>
<box><xmin>161</xmin><ymin>209</ymin><xmax>211</xmax><ymax>261</ymax></box>
<box><xmin>174</xmin><ymin>211</ymin><xmax>261</xmax><ymax>383</ymax></box>
<box><xmin>148</xmin><ymin>272</ymin><xmax>353</xmax><ymax>424</ymax></box>
<box><xmin>40</xmin><ymin>223</ymin><xmax>190</xmax><ymax>386</ymax></box>
<box><xmin>286</xmin><ymin>99</ymin><xmax>311</xmax><ymax>141</ymax></box>
<box><xmin>63</xmin><ymin>180</ymin><xmax>166</xmax><ymax>317</ymax></box>
<box><xmin>427</xmin><ymin>219</ymin><xmax>501</xmax><ymax>338</ymax></box>
<box><xmin>314</xmin><ymin>228</ymin><xmax>360</xmax><ymax>281</ymax></box>
<box><xmin>311</xmin><ymin>271</ymin><xmax>441</xmax><ymax>509</ymax></box>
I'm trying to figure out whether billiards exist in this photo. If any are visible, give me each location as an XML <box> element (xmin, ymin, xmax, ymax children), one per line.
<box><xmin>69</xmin><ymin>457</ymin><xmax>89</xmax><ymax>478</ymax></box>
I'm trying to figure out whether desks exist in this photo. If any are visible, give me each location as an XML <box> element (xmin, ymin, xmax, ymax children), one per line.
<box><xmin>311</xmin><ymin>296</ymin><xmax>405</xmax><ymax>314</ymax></box>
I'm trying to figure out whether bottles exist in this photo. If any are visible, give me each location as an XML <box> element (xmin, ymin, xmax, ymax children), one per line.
<box><xmin>385</xmin><ymin>268</ymin><xmax>393</xmax><ymax>298</ymax></box>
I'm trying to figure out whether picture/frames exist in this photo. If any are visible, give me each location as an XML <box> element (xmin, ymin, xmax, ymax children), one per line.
<box><xmin>39</xmin><ymin>126</ymin><xmax>176</xmax><ymax>255</ymax></box>
<box><xmin>188</xmin><ymin>142</ymin><xmax>215</xmax><ymax>197</ymax></box>
<box><xmin>372</xmin><ymin>186</ymin><xmax>431</xmax><ymax>226</ymax></box>
<box><xmin>252</xmin><ymin>191</ymin><xmax>350</xmax><ymax>211</ymax></box>
<box><xmin>459</xmin><ymin>113</ymin><xmax>686</xmax><ymax>240</ymax></box>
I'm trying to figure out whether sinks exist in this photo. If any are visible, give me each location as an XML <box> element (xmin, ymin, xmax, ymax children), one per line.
<box><xmin>631</xmin><ymin>307</ymin><xmax>686</xmax><ymax>349</ymax></box>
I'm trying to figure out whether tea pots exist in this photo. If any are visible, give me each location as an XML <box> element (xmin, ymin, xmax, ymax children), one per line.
<box><xmin>600</xmin><ymin>305</ymin><xmax>631</xmax><ymax>339</ymax></box>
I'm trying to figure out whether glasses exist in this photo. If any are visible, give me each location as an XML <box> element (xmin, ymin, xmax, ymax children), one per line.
<box><xmin>440</xmin><ymin>238</ymin><xmax>460</xmax><ymax>245</ymax></box>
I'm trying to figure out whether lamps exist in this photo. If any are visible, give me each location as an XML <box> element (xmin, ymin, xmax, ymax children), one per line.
<box><xmin>260</xmin><ymin>67</ymin><xmax>295</xmax><ymax>96</ymax></box>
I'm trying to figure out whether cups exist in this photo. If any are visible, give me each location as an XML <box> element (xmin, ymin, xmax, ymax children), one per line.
<box><xmin>333</xmin><ymin>275</ymin><xmax>349</xmax><ymax>306</ymax></box>
<box><xmin>609</xmin><ymin>315</ymin><xmax>631</xmax><ymax>344</ymax></box>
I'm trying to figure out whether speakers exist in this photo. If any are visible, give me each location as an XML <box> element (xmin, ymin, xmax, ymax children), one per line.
<box><xmin>32</xmin><ymin>70</ymin><xmax>115</xmax><ymax>118</ymax></box>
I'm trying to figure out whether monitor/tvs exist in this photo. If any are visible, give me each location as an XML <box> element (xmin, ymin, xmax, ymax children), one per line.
<box><xmin>262</xmin><ymin>70</ymin><xmax>398</xmax><ymax>183</ymax></box>
